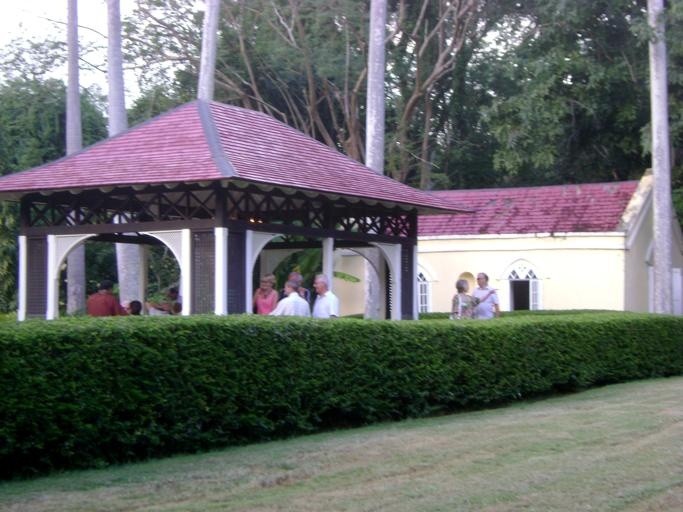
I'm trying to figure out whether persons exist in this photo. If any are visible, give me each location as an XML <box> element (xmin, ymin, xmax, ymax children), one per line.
<box><xmin>253</xmin><ymin>275</ymin><xmax>278</xmax><ymax>314</ymax></box>
<box><xmin>130</xmin><ymin>300</ymin><xmax>142</xmax><ymax>314</ymax></box>
<box><xmin>282</xmin><ymin>272</ymin><xmax>310</xmax><ymax>302</ymax></box>
<box><xmin>269</xmin><ymin>281</ymin><xmax>311</xmax><ymax>316</ymax></box>
<box><xmin>450</xmin><ymin>280</ymin><xmax>498</xmax><ymax>320</ymax></box>
<box><xmin>472</xmin><ymin>273</ymin><xmax>500</xmax><ymax>320</ymax></box>
<box><xmin>87</xmin><ymin>279</ymin><xmax>122</xmax><ymax>315</ymax></box>
<box><xmin>145</xmin><ymin>285</ymin><xmax>182</xmax><ymax>315</ymax></box>
<box><xmin>312</xmin><ymin>275</ymin><xmax>340</xmax><ymax>318</ymax></box>
